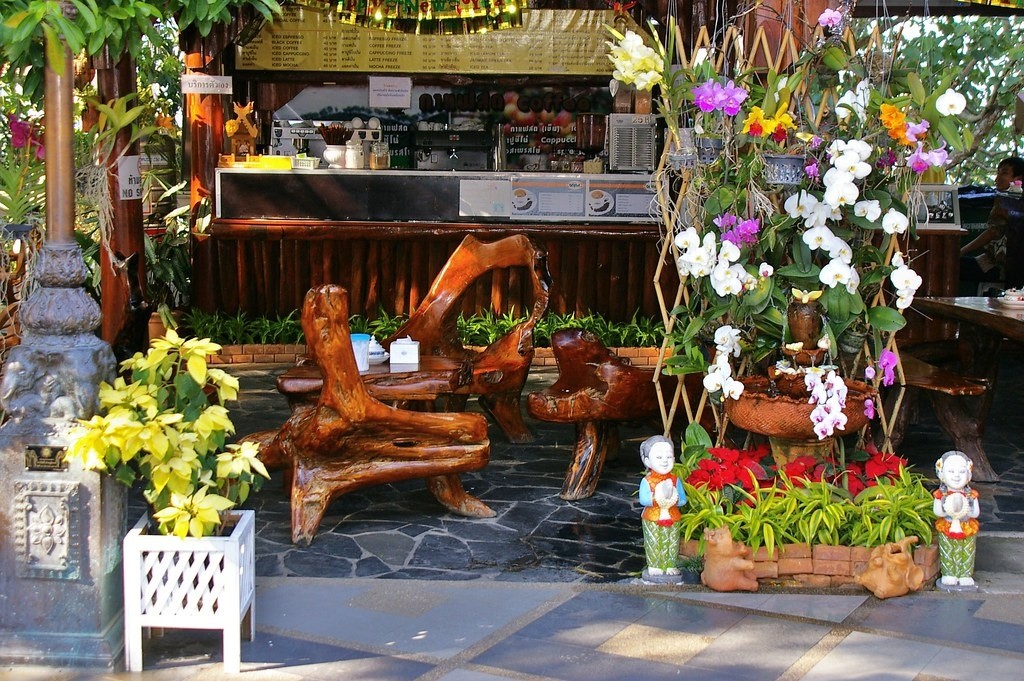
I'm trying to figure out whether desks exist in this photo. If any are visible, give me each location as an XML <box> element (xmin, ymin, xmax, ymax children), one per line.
<box><xmin>277</xmin><ymin>356</ymin><xmax>469</xmax><ymax>492</ymax></box>
<box><xmin>912</xmin><ymin>293</ymin><xmax>1024</xmax><ymax>422</ymax></box>
<box><xmin>875</xmin><ymin>218</ymin><xmax>967</xmax><ymax>343</ymax></box>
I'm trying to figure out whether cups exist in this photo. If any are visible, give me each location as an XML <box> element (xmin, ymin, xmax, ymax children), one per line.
<box><xmin>511</xmin><ymin>190</ymin><xmax>537</xmax><ymax>214</ymax></box>
<box><xmin>589</xmin><ymin>189</ymin><xmax>610</xmax><ymax>209</ymax></box>
<box><xmin>512</xmin><ymin>188</ymin><xmax>534</xmax><ymax>207</ymax></box>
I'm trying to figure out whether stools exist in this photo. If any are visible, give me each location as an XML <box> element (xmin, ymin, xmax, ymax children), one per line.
<box><xmin>976</xmin><ymin>281</ymin><xmax>1016</xmax><ymax>339</ymax></box>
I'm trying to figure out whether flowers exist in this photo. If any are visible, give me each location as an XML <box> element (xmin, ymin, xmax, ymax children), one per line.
<box><xmin>62</xmin><ymin>326</ymin><xmax>270</xmax><ymax>551</ymax></box>
<box><xmin>606</xmin><ymin>17</ymin><xmax>986</xmax><ymax>590</ymax></box>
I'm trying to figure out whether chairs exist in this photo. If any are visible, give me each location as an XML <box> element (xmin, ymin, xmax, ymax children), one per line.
<box><xmin>377</xmin><ymin>231</ymin><xmax>556</xmax><ymax>444</ymax></box>
<box><xmin>230</xmin><ymin>289</ymin><xmax>497</xmax><ymax>546</ymax></box>
<box><xmin>526</xmin><ymin>328</ymin><xmax>722</xmax><ymax>501</ymax></box>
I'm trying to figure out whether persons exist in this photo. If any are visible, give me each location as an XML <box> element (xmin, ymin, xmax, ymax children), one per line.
<box><xmin>960</xmin><ymin>156</ymin><xmax>1024</xmax><ymax>289</ymax></box>
<box><xmin>933</xmin><ymin>451</ymin><xmax>980</xmax><ymax>587</ymax></box>
<box><xmin>639</xmin><ymin>435</ymin><xmax>688</xmax><ymax>575</ymax></box>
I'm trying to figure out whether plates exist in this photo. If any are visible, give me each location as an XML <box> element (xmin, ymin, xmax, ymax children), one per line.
<box><xmin>997</xmin><ymin>296</ymin><xmax>1024</xmax><ymax>310</ymax></box>
<box><xmin>587</xmin><ymin>192</ymin><xmax>614</xmax><ymax>215</ymax></box>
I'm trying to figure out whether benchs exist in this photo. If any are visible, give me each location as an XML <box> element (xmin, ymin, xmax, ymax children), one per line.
<box><xmin>881</xmin><ymin>345</ymin><xmax>1001</xmax><ymax>482</ymax></box>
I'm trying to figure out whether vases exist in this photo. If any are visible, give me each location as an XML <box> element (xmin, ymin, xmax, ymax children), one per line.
<box><xmin>120</xmin><ymin>512</ymin><xmax>259</xmax><ymax>676</ymax></box>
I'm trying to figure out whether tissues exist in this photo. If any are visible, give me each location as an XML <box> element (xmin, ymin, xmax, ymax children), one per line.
<box><xmin>389</xmin><ymin>334</ymin><xmax>421</xmax><ymax>364</ymax></box>
<box><xmin>584</xmin><ymin>154</ymin><xmax>604</xmax><ymax>173</ymax></box>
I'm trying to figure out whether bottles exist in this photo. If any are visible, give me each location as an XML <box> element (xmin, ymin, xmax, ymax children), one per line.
<box><xmin>369</xmin><ymin>141</ymin><xmax>390</xmax><ymax>170</ymax></box>
<box><xmin>549</xmin><ymin>147</ymin><xmax>560</xmax><ymax>172</ymax></box>
<box><xmin>349</xmin><ymin>333</ymin><xmax>371</xmax><ymax>371</ymax></box>
<box><xmin>560</xmin><ymin>155</ymin><xmax>570</xmax><ymax>172</ymax></box>
<box><xmin>345</xmin><ymin>140</ymin><xmax>365</xmax><ymax>169</ymax></box>
